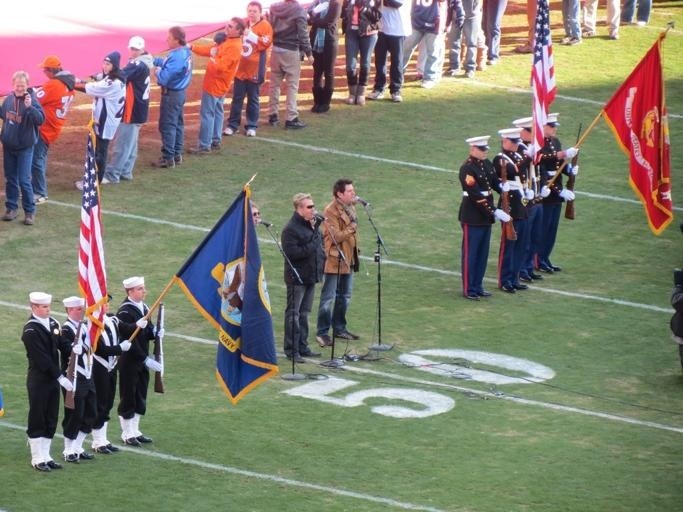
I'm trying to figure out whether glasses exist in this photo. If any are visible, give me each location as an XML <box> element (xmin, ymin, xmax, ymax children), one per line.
<box><xmin>253</xmin><ymin>212</ymin><xmax>261</xmax><ymax>216</ymax></box>
<box><xmin>307</xmin><ymin>205</ymin><xmax>314</xmax><ymax>209</ymax></box>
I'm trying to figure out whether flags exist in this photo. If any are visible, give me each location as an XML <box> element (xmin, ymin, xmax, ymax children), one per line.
<box><xmin>174</xmin><ymin>184</ymin><xmax>279</xmax><ymax>405</ymax></box>
<box><xmin>529</xmin><ymin>3</ymin><xmax>558</xmax><ymax>161</ymax></box>
<box><xmin>78</xmin><ymin>120</ymin><xmax>108</xmax><ymax>353</ymax></box>
<box><xmin>601</xmin><ymin>32</ymin><xmax>674</xmax><ymax>235</ymax></box>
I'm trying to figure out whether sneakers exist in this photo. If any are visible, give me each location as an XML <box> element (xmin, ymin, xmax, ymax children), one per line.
<box><xmin>516</xmin><ymin>17</ymin><xmax>648</xmax><ymax>54</ymax></box>
<box><xmin>346</xmin><ymin>47</ymin><xmax>498</xmax><ymax>103</ymax></box>
<box><xmin>75</xmin><ymin>151</ymin><xmax>181</xmax><ymax>190</ymax></box>
<box><xmin>4</xmin><ymin>193</ymin><xmax>47</xmax><ymax>226</ymax></box>
<box><xmin>188</xmin><ymin>100</ymin><xmax>331</xmax><ymax>154</ymax></box>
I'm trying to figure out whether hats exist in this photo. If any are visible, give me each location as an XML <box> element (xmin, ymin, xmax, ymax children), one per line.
<box><xmin>122</xmin><ymin>276</ymin><xmax>144</xmax><ymax>288</ymax></box>
<box><xmin>466</xmin><ymin>135</ymin><xmax>490</xmax><ymax>151</ymax></box>
<box><xmin>63</xmin><ymin>296</ymin><xmax>85</xmax><ymax>307</ymax></box>
<box><xmin>513</xmin><ymin>117</ymin><xmax>532</xmax><ymax>129</ymax></box>
<box><xmin>499</xmin><ymin>128</ymin><xmax>522</xmax><ymax>140</ymax></box>
<box><xmin>105</xmin><ymin>52</ymin><xmax>120</xmax><ymax>69</ymax></box>
<box><xmin>29</xmin><ymin>292</ymin><xmax>52</xmax><ymax>304</ymax></box>
<box><xmin>128</xmin><ymin>36</ymin><xmax>145</xmax><ymax>49</ymax></box>
<box><xmin>545</xmin><ymin>112</ymin><xmax>562</xmax><ymax>125</ymax></box>
<box><xmin>40</xmin><ymin>57</ymin><xmax>61</xmax><ymax>69</ymax></box>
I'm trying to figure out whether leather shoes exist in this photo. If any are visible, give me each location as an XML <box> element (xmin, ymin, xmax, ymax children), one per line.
<box><xmin>336</xmin><ymin>331</ymin><xmax>358</xmax><ymax>340</ymax></box>
<box><xmin>464</xmin><ymin>290</ymin><xmax>491</xmax><ymax>302</ymax></box>
<box><xmin>287</xmin><ymin>351</ymin><xmax>304</xmax><ymax>362</ymax></box>
<box><xmin>301</xmin><ymin>349</ymin><xmax>321</xmax><ymax>356</ymax></box>
<box><xmin>500</xmin><ymin>262</ymin><xmax>561</xmax><ymax>293</ymax></box>
<box><xmin>29</xmin><ymin>434</ymin><xmax>151</xmax><ymax>472</ymax></box>
<box><xmin>321</xmin><ymin>335</ymin><xmax>332</xmax><ymax>346</ymax></box>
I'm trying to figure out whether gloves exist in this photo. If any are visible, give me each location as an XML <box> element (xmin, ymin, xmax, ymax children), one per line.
<box><xmin>146</xmin><ymin>358</ymin><xmax>162</xmax><ymax>373</ymax></box>
<box><xmin>137</xmin><ymin>317</ymin><xmax>148</xmax><ymax>329</ymax></box>
<box><xmin>494</xmin><ymin>209</ymin><xmax>513</xmax><ymax>224</ymax></box>
<box><xmin>119</xmin><ymin>340</ymin><xmax>132</xmax><ymax>352</ymax></box>
<box><xmin>312</xmin><ymin>232</ymin><xmax>322</xmax><ymax>247</ymax></box>
<box><xmin>152</xmin><ymin>327</ymin><xmax>164</xmax><ymax>338</ymax></box>
<box><xmin>58</xmin><ymin>375</ymin><xmax>73</xmax><ymax>392</ymax></box>
<box><xmin>71</xmin><ymin>342</ymin><xmax>83</xmax><ymax>355</ymax></box>
<box><xmin>500</xmin><ymin>149</ymin><xmax>579</xmax><ymax>202</ymax></box>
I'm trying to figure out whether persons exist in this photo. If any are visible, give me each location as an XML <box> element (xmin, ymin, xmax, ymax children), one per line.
<box><xmin>403</xmin><ymin>1</ymin><xmax>507</xmax><ymax>89</ymax></box>
<box><xmin>83</xmin><ymin>294</ymin><xmax>148</xmax><ymax>454</ymax></box>
<box><xmin>515</xmin><ymin>1</ymin><xmax>651</xmax><ymax>53</ymax></box>
<box><xmin>0</xmin><ymin>56</ymin><xmax>76</xmax><ymax>225</ymax></box>
<box><xmin>22</xmin><ymin>292</ymin><xmax>82</xmax><ymax>472</ymax></box>
<box><xmin>59</xmin><ymin>296</ymin><xmax>98</xmax><ymax>463</ymax></box>
<box><xmin>281</xmin><ymin>180</ymin><xmax>359</xmax><ymax>363</ymax></box>
<box><xmin>457</xmin><ymin>113</ymin><xmax>580</xmax><ymax>300</ymax></box>
<box><xmin>74</xmin><ymin>26</ymin><xmax>192</xmax><ymax>190</ymax></box>
<box><xmin>188</xmin><ymin>0</ymin><xmax>315</xmax><ymax>153</ymax></box>
<box><xmin>116</xmin><ymin>276</ymin><xmax>165</xmax><ymax>447</ymax></box>
<box><xmin>305</xmin><ymin>0</ymin><xmax>413</xmax><ymax>114</ymax></box>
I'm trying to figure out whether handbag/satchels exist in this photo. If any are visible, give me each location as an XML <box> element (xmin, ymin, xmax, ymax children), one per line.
<box><xmin>359</xmin><ymin>18</ymin><xmax>378</xmax><ymax>37</ymax></box>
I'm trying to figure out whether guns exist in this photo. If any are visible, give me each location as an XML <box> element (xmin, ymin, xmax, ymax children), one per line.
<box><xmin>565</xmin><ymin>123</ymin><xmax>582</xmax><ymax>220</ymax></box>
<box><xmin>499</xmin><ymin>140</ymin><xmax>518</xmax><ymax>241</ymax></box>
<box><xmin>155</xmin><ymin>304</ymin><xmax>165</xmax><ymax>393</ymax></box>
<box><xmin>65</xmin><ymin>300</ymin><xmax>86</xmax><ymax>409</ymax></box>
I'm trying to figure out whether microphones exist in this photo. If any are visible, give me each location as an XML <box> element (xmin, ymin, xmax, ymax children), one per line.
<box><xmin>354</xmin><ymin>195</ymin><xmax>370</xmax><ymax>208</ymax></box>
<box><xmin>257</xmin><ymin>219</ymin><xmax>273</xmax><ymax>228</ymax></box>
<box><xmin>312</xmin><ymin>210</ymin><xmax>328</xmax><ymax>221</ymax></box>
